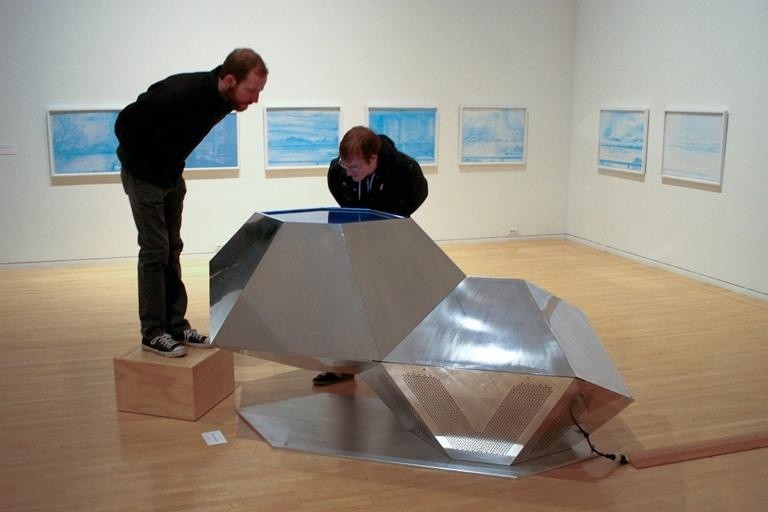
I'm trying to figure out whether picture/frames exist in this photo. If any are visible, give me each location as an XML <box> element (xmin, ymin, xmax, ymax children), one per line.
<box><xmin>367</xmin><ymin>106</ymin><xmax>440</xmax><ymax>168</ymax></box>
<box><xmin>262</xmin><ymin>105</ymin><xmax>342</xmax><ymax>171</ymax></box>
<box><xmin>459</xmin><ymin>105</ymin><xmax>529</xmax><ymax>167</ymax></box>
<box><xmin>183</xmin><ymin>111</ymin><xmax>239</xmax><ymax>172</ymax></box>
<box><xmin>662</xmin><ymin>109</ymin><xmax>728</xmax><ymax>188</ymax></box>
<box><xmin>46</xmin><ymin>106</ymin><xmax>122</xmax><ymax>179</ymax></box>
<box><xmin>597</xmin><ymin>107</ymin><xmax>649</xmax><ymax>175</ymax></box>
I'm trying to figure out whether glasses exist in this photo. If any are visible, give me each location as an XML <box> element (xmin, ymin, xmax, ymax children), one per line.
<box><xmin>337</xmin><ymin>158</ymin><xmax>368</xmax><ymax>170</ymax></box>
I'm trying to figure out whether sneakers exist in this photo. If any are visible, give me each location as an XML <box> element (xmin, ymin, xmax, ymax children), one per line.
<box><xmin>171</xmin><ymin>327</ymin><xmax>213</xmax><ymax>348</ymax></box>
<box><xmin>311</xmin><ymin>370</ymin><xmax>354</xmax><ymax>385</ymax></box>
<box><xmin>142</xmin><ymin>334</ymin><xmax>187</xmax><ymax>358</ymax></box>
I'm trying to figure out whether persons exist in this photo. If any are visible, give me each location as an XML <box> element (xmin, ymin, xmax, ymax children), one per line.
<box><xmin>112</xmin><ymin>47</ymin><xmax>271</xmax><ymax>359</ymax></box>
<box><xmin>312</xmin><ymin>124</ymin><xmax>429</xmax><ymax>385</ymax></box>
